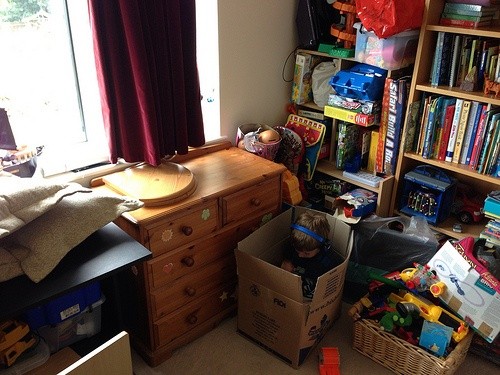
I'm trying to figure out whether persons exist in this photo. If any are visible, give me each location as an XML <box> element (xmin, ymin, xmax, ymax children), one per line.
<box><xmin>280</xmin><ymin>211</ymin><xmax>344</xmax><ymax>297</ymax></box>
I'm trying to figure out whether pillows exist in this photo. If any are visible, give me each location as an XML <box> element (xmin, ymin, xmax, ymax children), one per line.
<box><xmin>0</xmin><ymin>170</ymin><xmax>145</xmax><ymax>283</ymax></box>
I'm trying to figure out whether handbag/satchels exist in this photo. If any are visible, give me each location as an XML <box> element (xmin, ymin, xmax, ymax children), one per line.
<box><xmin>342</xmin><ymin>214</ymin><xmax>440</xmax><ymax>302</ymax></box>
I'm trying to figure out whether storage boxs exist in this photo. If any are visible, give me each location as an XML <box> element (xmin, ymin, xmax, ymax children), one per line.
<box><xmin>234</xmin><ymin>206</ymin><xmax>354</xmax><ymax>369</ymax></box>
<box><xmin>324</xmin><ymin>105</ymin><xmax>380</xmax><ymax>127</ymax></box>
<box><xmin>353</xmin><ymin>22</ymin><xmax>420</xmax><ymax>72</ymax></box>
<box><xmin>35</xmin><ymin>294</ymin><xmax>106</xmax><ymax>355</ymax></box>
<box><xmin>291</xmin><ymin>52</ymin><xmax>322</xmax><ymax>105</ymax></box>
<box><xmin>375</xmin><ymin>77</ymin><xmax>409</xmax><ymax>175</ymax></box>
<box><xmin>332</xmin><ymin>188</ymin><xmax>378</xmax><ymax>225</ymax></box>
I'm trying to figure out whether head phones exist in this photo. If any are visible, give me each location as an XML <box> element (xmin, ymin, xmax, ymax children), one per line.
<box><xmin>292</xmin><ymin>224</ymin><xmax>332</xmax><ymax>254</ymax></box>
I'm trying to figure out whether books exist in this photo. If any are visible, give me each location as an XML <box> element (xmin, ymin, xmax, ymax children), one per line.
<box><xmin>439</xmin><ymin>0</ymin><xmax>500</xmax><ymax>30</ymax></box>
<box><xmin>479</xmin><ymin>193</ymin><xmax>500</xmax><ymax>248</ymax></box>
<box><xmin>429</xmin><ymin>31</ymin><xmax>500</xmax><ymax>94</ymax></box>
<box><xmin>407</xmin><ymin>96</ymin><xmax>500</xmax><ymax>177</ymax></box>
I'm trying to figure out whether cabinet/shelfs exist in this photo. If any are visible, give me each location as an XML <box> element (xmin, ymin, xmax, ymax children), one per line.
<box><xmin>391</xmin><ymin>0</ymin><xmax>500</xmax><ymax>241</ymax></box>
<box><xmin>91</xmin><ymin>141</ymin><xmax>288</xmax><ymax>368</ymax></box>
<box><xmin>294</xmin><ymin>48</ymin><xmax>395</xmax><ymax>218</ymax></box>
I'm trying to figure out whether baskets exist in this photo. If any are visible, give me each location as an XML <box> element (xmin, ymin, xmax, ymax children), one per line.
<box><xmin>349</xmin><ymin>283</ymin><xmax>474</xmax><ymax>375</ymax></box>
